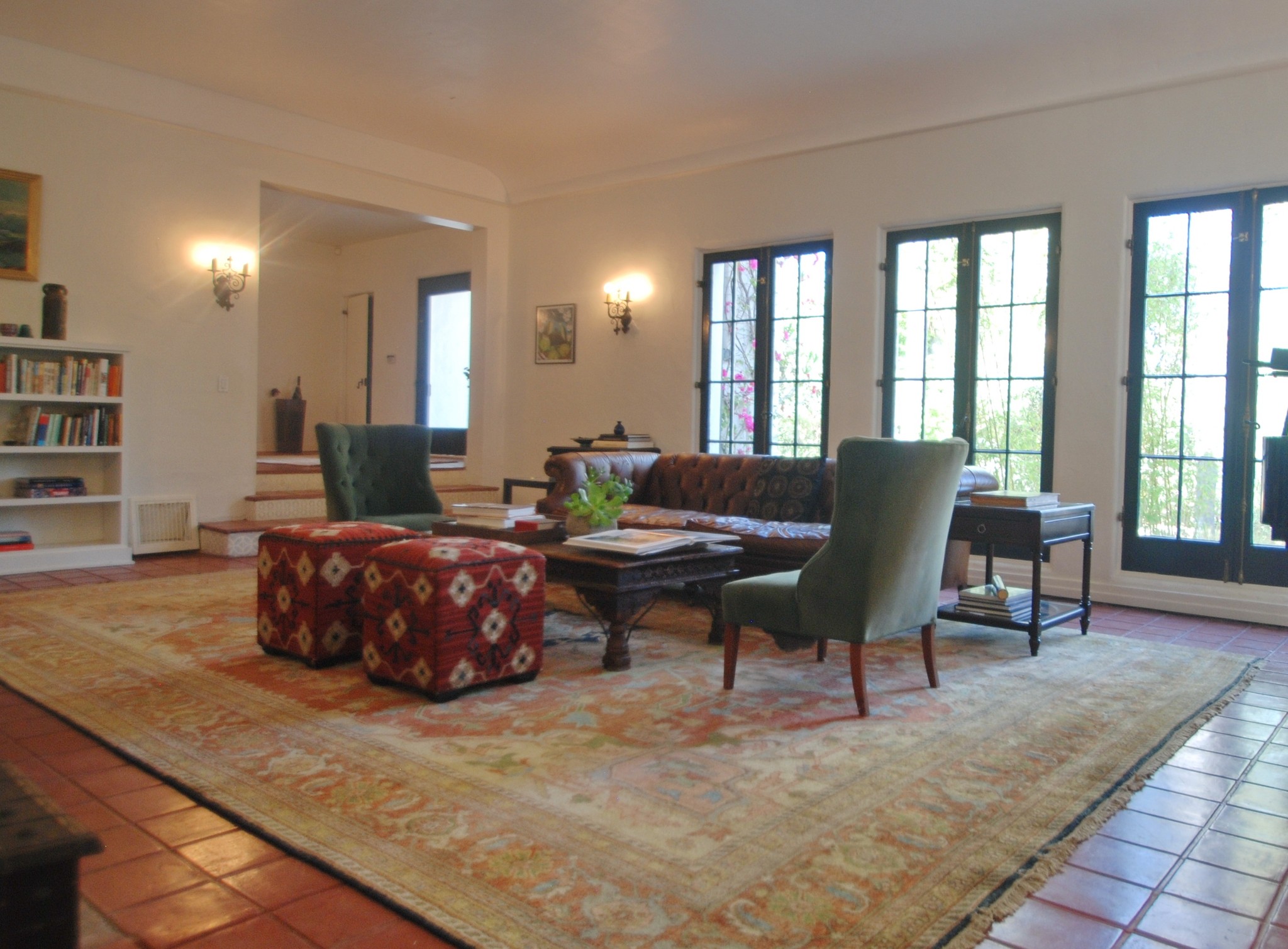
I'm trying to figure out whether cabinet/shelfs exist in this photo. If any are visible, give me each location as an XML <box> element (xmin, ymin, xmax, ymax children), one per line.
<box><xmin>0</xmin><ymin>336</ymin><xmax>134</xmax><ymax>576</ymax></box>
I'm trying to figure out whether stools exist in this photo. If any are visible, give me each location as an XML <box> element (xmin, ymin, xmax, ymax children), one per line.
<box><xmin>364</xmin><ymin>536</ymin><xmax>544</xmax><ymax>704</ymax></box>
<box><xmin>257</xmin><ymin>521</ymin><xmax>429</xmax><ymax>670</ymax></box>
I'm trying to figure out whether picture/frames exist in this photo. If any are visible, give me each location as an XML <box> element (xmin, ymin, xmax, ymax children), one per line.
<box><xmin>0</xmin><ymin>168</ymin><xmax>43</xmax><ymax>281</ymax></box>
<box><xmin>535</xmin><ymin>303</ymin><xmax>575</xmax><ymax>364</ymax></box>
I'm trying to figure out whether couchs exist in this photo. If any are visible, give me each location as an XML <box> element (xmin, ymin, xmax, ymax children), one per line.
<box><xmin>535</xmin><ymin>436</ymin><xmax>998</xmax><ymax>591</ymax></box>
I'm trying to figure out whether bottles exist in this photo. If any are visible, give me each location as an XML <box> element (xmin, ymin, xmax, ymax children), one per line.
<box><xmin>614</xmin><ymin>420</ymin><xmax>625</xmax><ymax>434</ymax></box>
<box><xmin>42</xmin><ymin>283</ymin><xmax>69</xmax><ymax>341</ymax></box>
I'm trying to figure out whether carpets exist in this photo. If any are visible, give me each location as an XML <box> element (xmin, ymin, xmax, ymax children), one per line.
<box><xmin>0</xmin><ymin>567</ymin><xmax>1267</xmax><ymax>949</ymax></box>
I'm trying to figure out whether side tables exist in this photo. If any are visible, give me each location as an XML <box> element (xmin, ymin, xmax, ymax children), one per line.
<box><xmin>503</xmin><ymin>477</ymin><xmax>556</xmax><ymax>504</ymax></box>
<box><xmin>937</xmin><ymin>500</ymin><xmax>1095</xmax><ymax>656</ymax></box>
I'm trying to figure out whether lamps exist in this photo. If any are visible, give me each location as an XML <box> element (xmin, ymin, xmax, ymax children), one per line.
<box><xmin>192</xmin><ymin>242</ymin><xmax>254</xmax><ymax>312</ymax></box>
<box><xmin>604</xmin><ymin>274</ymin><xmax>653</xmax><ymax>335</ymax></box>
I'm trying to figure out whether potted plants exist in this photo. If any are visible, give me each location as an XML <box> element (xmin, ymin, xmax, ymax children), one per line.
<box><xmin>563</xmin><ymin>467</ymin><xmax>633</xmax><ymax>538</ymax></box>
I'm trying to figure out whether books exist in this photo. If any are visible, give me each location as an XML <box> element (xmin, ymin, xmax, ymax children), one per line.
<box><xmin>0</xmin><ymin>354</ymin><xmax>121</xmax><ymax>397</ymax></box>
<box><xmin>0</xmin><ymin>531</ymin><xmax>33</xmax><ymax>552</ymax></box>
<box><xmin>452</xmin><ymin>503</ymin><xmax>566</xmax><ymax>531</ymax></box>
<box><xmin>562</xmin><ymin>529</ymin><xmax>741</xmax><ymax>556</ymax></box>
<box><xmin>16</xmin><ymin>476</ymin><xmax>88</xmax><ymax>498</ymax></box>
<box><xmin>591</xmin><ymin>434</ymin><xmax>653</xmax><ymax>448</ymax></box>
<box><xmin>971</xmin><ymin>491</ymin><xmax>1060</xmax><ymax>507</ymax></box>
<box><xmin>17</xmin><ymin>407</ymin><xmax>122</xmax><ymax>446</ymax></box>
<box><xmin>954</xmin><ymin>585</ymin><xmax>1032</xmax><ymax>617</ymax></box>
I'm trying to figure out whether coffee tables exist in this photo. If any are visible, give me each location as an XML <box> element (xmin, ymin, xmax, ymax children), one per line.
<box><xmin>432</xmin><ymin>513</ymin><xmax>744</xmax><ymax>672</ymax></box>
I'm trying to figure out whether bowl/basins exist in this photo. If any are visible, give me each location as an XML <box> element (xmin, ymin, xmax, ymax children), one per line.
<box><xmin>0</xmin><ymin>323</ymin><xmax>18</xmax><ymax>337</ymax></box>
<box><xmin>571</xmin><ymin>438</ymin><xmax>596</xmax><ymax>446</ymax></box>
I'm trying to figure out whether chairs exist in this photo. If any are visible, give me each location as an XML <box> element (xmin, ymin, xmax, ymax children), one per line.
<box><xmin>315</xmin><ymin>422</ymin><xmax>452</xmax><ymax>531</ymax></box>
<box><xmin>720</xmin><ymin>437</ymin><xmax>970</xmax><ymax>716</ymax></box>
